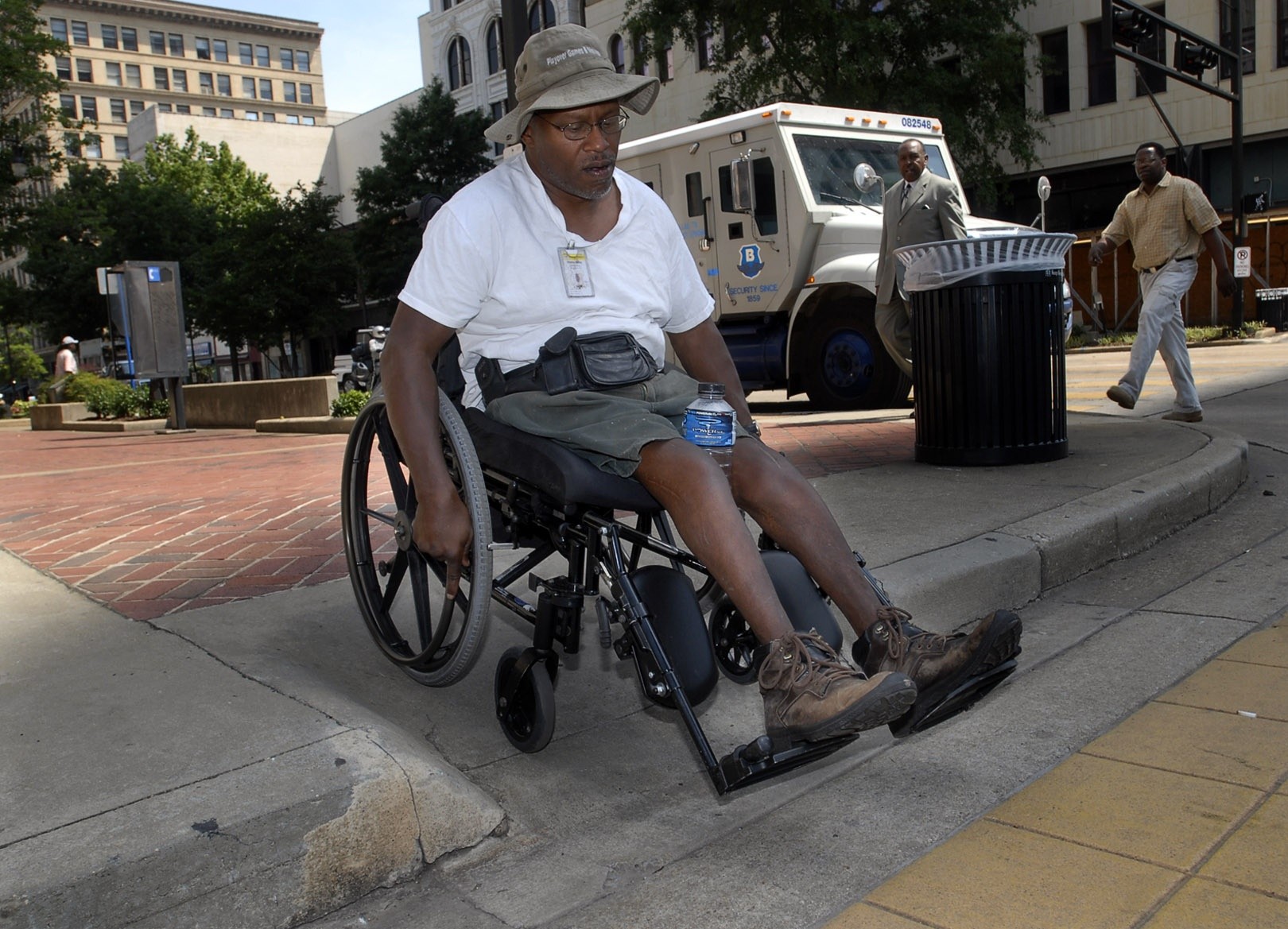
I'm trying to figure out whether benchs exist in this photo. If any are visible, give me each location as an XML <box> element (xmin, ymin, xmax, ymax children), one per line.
<box><xmin>331</xmin><ymin>355</ymin><xmax>353</xmax><ymax>382</ymax></box>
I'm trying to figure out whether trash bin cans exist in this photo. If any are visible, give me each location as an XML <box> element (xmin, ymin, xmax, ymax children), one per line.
<box><xmin>893</xmin><ymin>232</ymin><xmax>1079</xmax><ymax>468</ymax></box>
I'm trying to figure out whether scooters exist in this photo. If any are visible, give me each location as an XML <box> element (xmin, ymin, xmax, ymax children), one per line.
<box><xmin>341</xmin><ymin>324</ymin><xmax>392</xmax><ymax>394</ymax></box>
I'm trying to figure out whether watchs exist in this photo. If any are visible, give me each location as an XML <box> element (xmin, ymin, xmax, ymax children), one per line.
<box><xmin>741</xmin><ymin>419</ymin><xmax>762</xmax><ymax>437</ymax></box>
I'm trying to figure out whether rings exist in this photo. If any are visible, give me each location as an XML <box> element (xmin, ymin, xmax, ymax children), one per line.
<box><xmin>1094</xmin><ymin>259</ymin><xmax>1097</xmax><ymax>262</ymax></box>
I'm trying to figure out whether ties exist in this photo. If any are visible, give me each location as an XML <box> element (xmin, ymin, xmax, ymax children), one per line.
<box><xmin>901</xmin><ymin>185</ymin><xmax>911</xmax><ymax>214</ymax></box>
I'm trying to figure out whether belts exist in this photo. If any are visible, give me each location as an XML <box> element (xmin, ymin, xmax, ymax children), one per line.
<box><xmin>1140</xmin><ymin>255</ymin><xmax>1197</xmax><ymax>273</ymax></box>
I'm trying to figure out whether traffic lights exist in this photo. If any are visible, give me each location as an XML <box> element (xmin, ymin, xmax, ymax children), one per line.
<box><xmin>1174</xmin><ymin>40</ymin><xmax>1219</xmax><ymax>75</ymax></box>
<box><xmin>1115</xmin><ymin>7</ymin><xmax>1157</xmax><ymax>47</ymax></box>
<box><xmin>1245</xmin><ymin>191</ymin><xmax>1268</xmax><ymax>213</ymax></box>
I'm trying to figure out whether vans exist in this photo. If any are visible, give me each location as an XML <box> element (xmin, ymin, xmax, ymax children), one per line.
<box><xmin>613</xmin><ymin>101</ymin><xmax>1074</xmax><ymax>411</ymax></box>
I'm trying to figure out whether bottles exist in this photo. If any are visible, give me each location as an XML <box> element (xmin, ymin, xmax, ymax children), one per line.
<box><xmin>683</xmin><ymin>382</ymin><xmax>736</xmax><ymax>492</ymax></box>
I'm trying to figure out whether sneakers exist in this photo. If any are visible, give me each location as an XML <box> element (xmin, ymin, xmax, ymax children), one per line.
<box><xmin>851</xmin><ymin>606</ymin><xmax>1024</xmax><ymax>739</ymax></box>
<box><xmin>1161</xmin><ymin>409</ymin><xmax>1203</xmax><ymax>422</ymax></box>
<box><xmin>753</xmin><ymin>626</ymin><xmax>917</xmax><ymax>745</ymax></box>
<box><xmin>1106</xmin><ymin>386</ymin><xmax>1136</xmax><ymax>409</ymax></box>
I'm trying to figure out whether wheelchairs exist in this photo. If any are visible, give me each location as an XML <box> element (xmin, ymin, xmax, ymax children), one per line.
<box><xmin>338</xmin><ymin>332</ymin><xmax>1022</xmax><ymax>800</ymax></box>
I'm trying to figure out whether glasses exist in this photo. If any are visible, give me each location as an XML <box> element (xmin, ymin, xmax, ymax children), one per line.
<box><xmin>534</xmin><ymin>106</ymin><xmax>630</xmax><ymax>140</ymax></box>
<box><xmin>1132</xmin><ymin>156</ymin><xmax>1163</xmax><ymax>165</ymax></box>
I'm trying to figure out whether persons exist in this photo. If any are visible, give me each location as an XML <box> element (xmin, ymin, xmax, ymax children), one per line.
<box><xmin>1087</xmin><ymin>141</ymin><xmax>1237</xmax><ymax>422</ymax></box>
<box><xmin>380</xmin><ymin>24</ymin><xmax>1023</xmax><ymax>755</ymax></box>
<box><xmin>875</xmin><ymin>139</ymin><xmax>971</xmax><ymax>418</ymax></box>
<box><xmin>369</xmin><ymin>326</ymin><xmax>386</xmax><ymax>392</ymax></box>
<box><xmin>54</xmin><ymin>337</ymin><xmax>78</xmax><ymax>377</ymax></box>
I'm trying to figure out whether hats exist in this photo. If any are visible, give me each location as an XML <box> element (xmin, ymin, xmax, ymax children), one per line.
<box><xmin>483</xmin><ymin>22</ymin><xmax>660</xmax><ymax>144</ymax></box>
<box><xmin>62</xmin><ymin>336</ymin><xmax>79</xmax><ymax>345</ymax></box>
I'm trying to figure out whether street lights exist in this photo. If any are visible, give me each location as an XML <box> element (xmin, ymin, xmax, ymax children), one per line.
<box><xmin>1252</xmin><ymin>176</ymin><xmax>1273</xmax><ymax>288</ymax></box>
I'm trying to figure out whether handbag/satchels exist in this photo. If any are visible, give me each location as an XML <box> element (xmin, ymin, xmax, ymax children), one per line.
<box><xmin>503</xmin><ymin>326</ymin><xmax>658</xmax><ymax>396</ymax></box>
